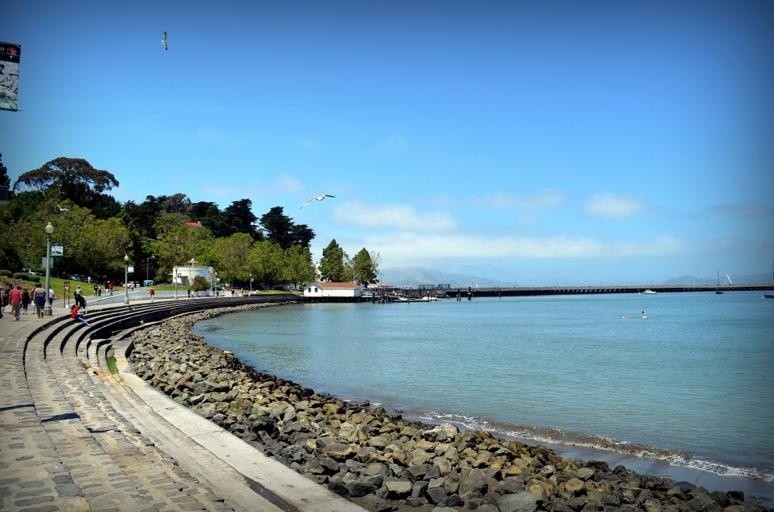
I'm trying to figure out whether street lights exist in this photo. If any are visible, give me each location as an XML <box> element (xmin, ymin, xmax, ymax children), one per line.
<box><xmin>44</xmin><ymin>221</ymin><xmax>54</xmax><ymax>315</ymax></box>
<box><xmin>208</xmin><ymin>266</ymin><xmax>220</xmax><ymax>297</ymax></box>
<box><xmin>124</xmin><ymin>254</ymin><xmax>129</xmax><ymax>304</ymax></box>
<box><xmin>147</xmin><ymin>254</ymin><xmax>155</xmax><ymax>280</ymax></box>
<box><xmin>250</xmin><ymin>274</ymin><xmax>254</xmax><ymax>290</ymax></box>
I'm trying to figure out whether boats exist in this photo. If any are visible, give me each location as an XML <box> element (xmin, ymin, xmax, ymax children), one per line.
<box><xmin>644</xmin><ymin>290</ymin><xmax>657</xmax><ymax>294</ymax></box>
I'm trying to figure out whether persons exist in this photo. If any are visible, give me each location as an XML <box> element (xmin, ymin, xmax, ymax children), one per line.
<box><xmin>87</xmin><ymin>276</ymin><xmax>91</xmax><ymax>285</ymax></box>
<box><xmin>75</xmin><ymin>286</ymin><xmax>81</xmax><ymax>296</ymax></box>
<box><xmin>93</xmin><ymin>283</ymin><xmax>98</xmax><ymax>297</ymax></box>
<box><xmin>108</xmin><ymin>282</ymin><xmax>112</xmax><ymax>295</ymax></box>
<box><xmin>0</xmin><ymin>284</ymin><xmax>54</xmax><ymax>320</ymax></box>
<box><xmin>148</xmin><ymin>288</ymin><xmax>154</xmax><ymax>302</ymax></box>
<box><xmin>130</xmin><ymin>283</ymin><xmax>133</xmax><ymax>291</ymax></box>
<box><xmin>73</xmin><ymin>292</ymin><xmax>85</xmax><ymax>307</ymax></box>
<box><xmin>105</xmin><ymin>281</ymin><xmax>108</xmax><ymax>294</ymax></box>
<box><xmin>71</xmin><ymin>305</ymin><xmax>88</xmax><ymax>325</ymax></box>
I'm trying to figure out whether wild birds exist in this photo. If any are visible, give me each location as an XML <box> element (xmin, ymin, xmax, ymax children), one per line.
<box><xmin>160</xmin><ymin>31</ymin><xmax>169</xmax><ymax>52</ymax></box>
<box><xmin>300</xmin><ymin>193</ymin><xmax>335</xmax><ymax>210</ymax></box>
